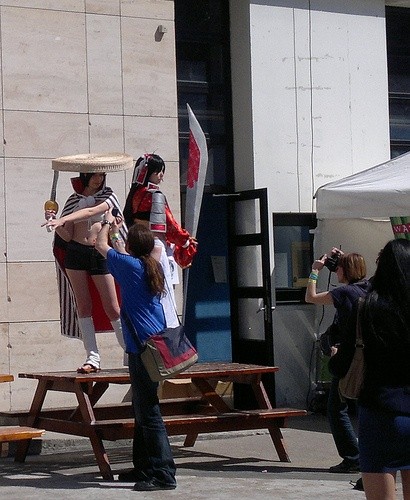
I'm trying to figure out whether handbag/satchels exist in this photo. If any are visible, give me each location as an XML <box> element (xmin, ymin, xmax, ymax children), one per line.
<box><xmin>319</xmin><ymin>322</ymin><xmax>340</xmax><ymax>357</ymax></box>
<box><xmin>338</xmin><ymin>296</ymin><xmax>366</xmax><ymax>401</ymax></box>
<box><xmin>138</xmin><ymin>324</ymin><xmax>200</xmax><ymax>384</ymax></box>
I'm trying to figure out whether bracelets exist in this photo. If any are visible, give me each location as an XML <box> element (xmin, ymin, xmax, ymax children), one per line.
<box><xmin>307</xmin><ymin>272</ymin><xmax>319</xmax><ymax>283</ymax></box>
<box><xmin>101</xmin><ymin>220</ymin><xmax>112</xmax><ymax>229</ymax></box>
<box><xmin>109</xmin><ymin>234</ymin><xmax>124</xmax><ymax>246</ymax></box>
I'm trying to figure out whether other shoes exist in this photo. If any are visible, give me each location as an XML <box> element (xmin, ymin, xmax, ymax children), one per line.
<box><xmin>329</xmin><ymin>452</ymin><xmax>361</xmax><ymax>474</ymax></box>
<box><xmin>133</xmin><ymin>476</ymin><xmax>177</xmax><ymax>491</ymax></box>
<box><xmin>118</xmin><ymin>469</ymin><xmax>153</xmax><ymax>483</ymax></box>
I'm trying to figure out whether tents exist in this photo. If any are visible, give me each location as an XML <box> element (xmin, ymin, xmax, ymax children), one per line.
<box><xmin>303</xmin><ymin>151</ymin><xmax>410</xmax><ymax>409</ymax></box>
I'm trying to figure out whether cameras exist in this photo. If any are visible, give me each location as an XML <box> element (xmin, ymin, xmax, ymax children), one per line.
<box><xmin>320</xmin><ymin>255</ymin><xmax>340</xmax><ymax>272</ymax></box>
<box><xmin>112</xmin><ymin>207</ymin><xmax>119</xmax><ymax>218</ymax></box>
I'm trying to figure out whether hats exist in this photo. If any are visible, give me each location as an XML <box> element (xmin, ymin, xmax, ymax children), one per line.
<box><xmin>51</xmin><ymin>152</ymin><xmax>133</xmax><ymax>173</ymax></box>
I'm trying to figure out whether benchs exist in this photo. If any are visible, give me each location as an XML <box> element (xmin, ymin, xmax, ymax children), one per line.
<box><xmin>0</xmin><ymin>425</ymin><xmax>45</xmax><ymax>444</ymax></box>
<box><xmin>94</xmin><ymin>408</ymin><xmax>307</xmax><ymax>433</ymax></box>
<box><xmin>0</xmin><ymin>396</ymin><xmax>207</xmax><ymax>418</ymax></box>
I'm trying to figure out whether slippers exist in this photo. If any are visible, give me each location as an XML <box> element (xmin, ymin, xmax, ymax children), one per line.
<box><xmin>77</xmin><ymin>363</ymin><xmax>102</xmax><ymax>375</ymax></box>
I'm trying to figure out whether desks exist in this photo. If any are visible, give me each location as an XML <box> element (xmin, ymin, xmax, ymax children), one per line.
<box><xmin>14</xmin><ymin>362</ymin><xmax>292</xmax><ymax>480</ymax></box>
<box><xmin>0</xmin><ymin>374</ymin><xmax>13</xmax><ymax>384</ymax></box>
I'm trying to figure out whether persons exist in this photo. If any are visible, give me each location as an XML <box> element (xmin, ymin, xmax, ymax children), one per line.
<box><xmin>123</xmin><ymin>154</ymin><xmax>198</xmax><ymax>284</ymax></box>
<box><xmin>96</xmin><ymin>206</ymin><xmax>176</xmax><ymax>491</ymax></box>
<box><xmin>306</xmin><ymin>247</ymin><xmax>374</xmax><ymax>473</ymax></box>
<box><xmin>358</xmin><ymin>237</ymin><xmax>410</xmax><ymax>500</ymax></box>
<box><xmin>41</xmin><ymin>172</ymin><xmax>128</xmax><ymax>373</ymax></box>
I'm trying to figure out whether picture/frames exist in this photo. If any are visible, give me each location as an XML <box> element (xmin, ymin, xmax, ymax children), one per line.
<box><xmin>291</xmin><ymin>239</ymin><xmax>314</xmax><ymax>288</ymax></box>
